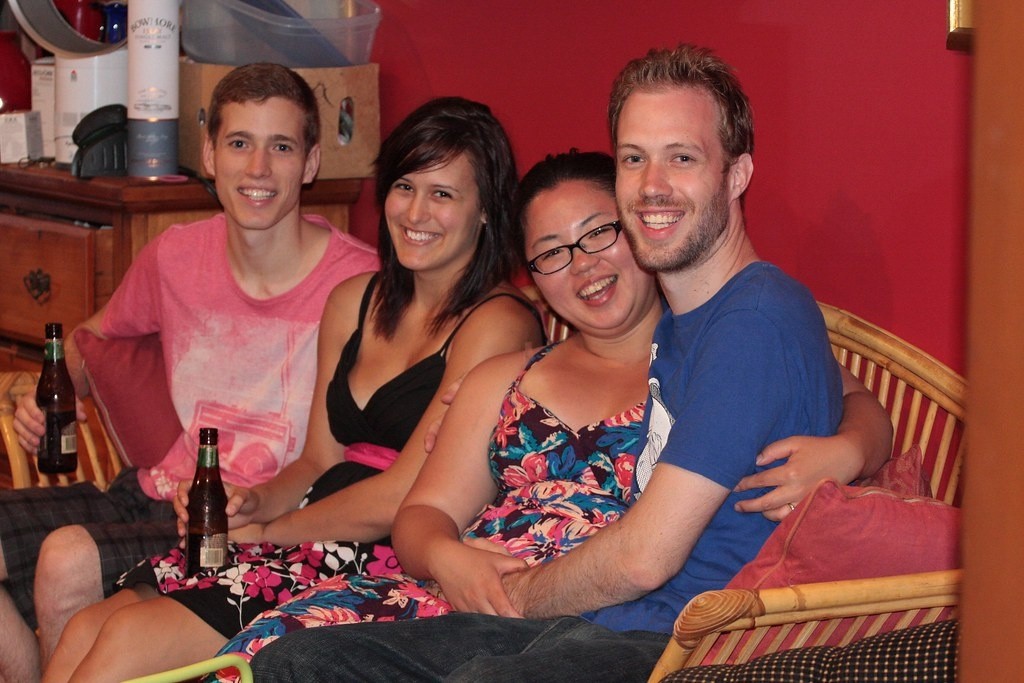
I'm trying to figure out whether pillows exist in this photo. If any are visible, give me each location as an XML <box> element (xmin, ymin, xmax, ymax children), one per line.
<box><xmin>688</xmin><ymin>473</ymin><xmax>965</xmax><ymax>666</ymax></box>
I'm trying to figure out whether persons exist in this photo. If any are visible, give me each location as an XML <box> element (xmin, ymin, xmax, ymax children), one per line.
<box><xmin>247</xmin><ymin>44</ymin><xmax>843</xmax><ymax>683</ymax></box>
<box><xmin>44</xmin><ymin>98</ymin><xmax>547</xmax><ymax>682</ymax></box>
<box><xmin>0</xmin><ymin>63</ymin><xmax>382</xmax><ymax>683</ymax></box>
<box><xmin>208</xmin><ymin>150</ymin><xmax>893</xmax><ymax>683</ymax></box>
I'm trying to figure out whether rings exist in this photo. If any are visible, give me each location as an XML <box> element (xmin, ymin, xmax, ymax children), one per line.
<box><xmin>788</xmin><ymin>503</ymin><xmax>795</xmax><ymax>511</ymax></box>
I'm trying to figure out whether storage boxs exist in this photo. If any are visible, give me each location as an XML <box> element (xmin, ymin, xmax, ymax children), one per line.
<box><xmin>182</xmin><ymin>0</ymin><xmax>382</xmax><ymax>69</ymax></box>
<box><xmin>30</xmin><ymin>54</ymin><xmax>56</xmax><ymax>161</ymax></box>
<box><xmin>0</xmin><ymin>109</ymin><xmax>43</xmax><ymax>164</ymax></box>
<box><xmin>177</xmin><ymin>52</ymin><xmax>379</xmax><ymax>180</ymax></box>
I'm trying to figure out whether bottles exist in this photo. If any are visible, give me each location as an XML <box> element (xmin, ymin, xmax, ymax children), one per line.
<box><xmin>35</xmin><ymin>320</ymin><xmax>79</xmax><ymax>474</ymax></box>
<box><xmin>184</xmin><ymin>427</ymin><xmax>229</xmax><ymax>580</ymax></box>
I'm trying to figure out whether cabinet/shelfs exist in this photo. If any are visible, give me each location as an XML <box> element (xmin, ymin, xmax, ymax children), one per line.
<box><xmin>0</xmin><ymin>159</ymin><xmax>360</xmax><ymax>369</ymax></box>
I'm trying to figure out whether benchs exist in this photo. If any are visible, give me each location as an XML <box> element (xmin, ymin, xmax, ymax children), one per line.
<box><xmin>503</xmin><ymin>278</ymin><xmax>969</xmax><ymax>682</ymax></box>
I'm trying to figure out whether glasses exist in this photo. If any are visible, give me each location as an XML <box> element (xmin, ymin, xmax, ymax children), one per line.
<box><xmin>526</xmin><ymin>217</ymin><xmax>624</xmax><ymax>275</ymax></box>
